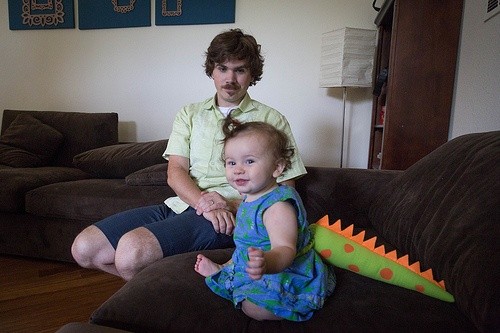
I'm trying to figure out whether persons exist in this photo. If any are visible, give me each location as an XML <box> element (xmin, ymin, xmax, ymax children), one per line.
<box><xmin>71</xmin><ymin>29</ymin><xmax>308</xmax><ymax>281</ymax></box>
<box><xmin>195</xmin><ymin>110</ymin><xmax>336</xmax><ymax>322</ymax></box>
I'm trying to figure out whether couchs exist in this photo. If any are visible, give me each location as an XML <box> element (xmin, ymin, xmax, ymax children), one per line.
<box><xmin>54</xmin><ymin>131</ymin><xmax>500</xmax><ymax>333</ymax></box>
<box><xmin>0</xmin><ymin>108</ymin><xmax>177</xmax><ymax>265</ymax></box>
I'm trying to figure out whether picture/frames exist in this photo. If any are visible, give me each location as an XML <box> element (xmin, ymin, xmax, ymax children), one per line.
<box><xmin>482</xmin><ymin>0</ymin><xmax>500</xmax><ymax>22</ymax></box>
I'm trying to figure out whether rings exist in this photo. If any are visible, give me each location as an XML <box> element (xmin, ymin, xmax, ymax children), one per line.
<box><xmin>211</xmin><ymin>200</ymin><xmax>214</xmax><ymax>204</ymax></box>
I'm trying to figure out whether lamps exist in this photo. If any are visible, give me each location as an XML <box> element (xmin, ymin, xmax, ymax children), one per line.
<box><xmin>319</xmin><ymin>26</ymin><xmax>377</xmax><ymax>168</ymax></box>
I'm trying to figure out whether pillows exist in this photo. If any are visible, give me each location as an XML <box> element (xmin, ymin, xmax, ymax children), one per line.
<box><xmin>72</xmin><ymin>138</ymin><xmax>169</xmax><ymax>178</ymax></box>
<box><xmin>0</xmin><ymin>113</ymin><xmax>77</xmax><ymax>169</ymax></box>
<box><xmin>126</xmin><ymin>163</ymin><xmax>169</xmax><ymax>187</ymax></box>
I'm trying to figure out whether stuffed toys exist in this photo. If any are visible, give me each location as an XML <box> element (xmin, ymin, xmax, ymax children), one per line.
<box><xmin>308</xmin><ymin>215</ymin><xmax>454</xmax><ymax>302</ymax></box>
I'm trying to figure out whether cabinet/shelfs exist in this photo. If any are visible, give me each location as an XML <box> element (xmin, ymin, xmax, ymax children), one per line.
<box><xmin>367</xmin><ymin>0</ymin><xmax>464</xmax><ymax>171</ymax></box>
